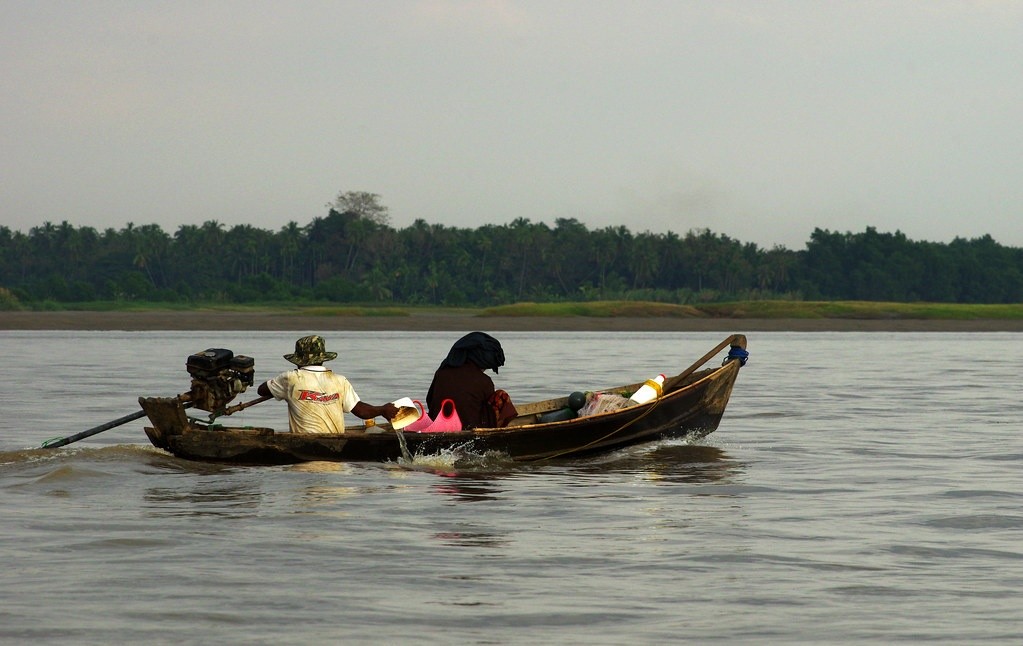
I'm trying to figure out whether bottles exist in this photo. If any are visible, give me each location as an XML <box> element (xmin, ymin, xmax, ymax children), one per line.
<box><xmin>628</xmin><ymin>374</ymin><xmax>666</xmax><ymax>406</ymax></box>
<box><xmin>362</xmin><ymin>417</ymin><xmax>386</xmax><ymax>433</ymax></box>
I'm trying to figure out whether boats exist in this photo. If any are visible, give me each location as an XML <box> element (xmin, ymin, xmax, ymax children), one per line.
<box><xmin>136</xmin><ymin>332</ymin><xmax>749</xmax><ymax>464</ymax></box>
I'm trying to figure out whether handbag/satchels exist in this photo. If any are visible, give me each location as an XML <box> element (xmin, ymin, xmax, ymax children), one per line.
<box><xmin>404</xmin><ymin>398</ymin><xmax>462</xmax><ymax>432</ymax></box>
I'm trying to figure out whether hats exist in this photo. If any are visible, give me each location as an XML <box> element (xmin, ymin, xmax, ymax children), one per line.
<box><xmin>283</xmin><ymin>335</ymin><xmax>338</xmax><ymax>366</ymax></box>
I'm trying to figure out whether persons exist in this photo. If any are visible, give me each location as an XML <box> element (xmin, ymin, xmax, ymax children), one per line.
<box><xmin>429</xmin><ymin>332</ymin><xmax>519</xmax><ymax>431</ymax></box>
<box><xmin>258</xmin><ymin>335</ymin><xmax>400</xmax><ymax>435</ymax></box>
